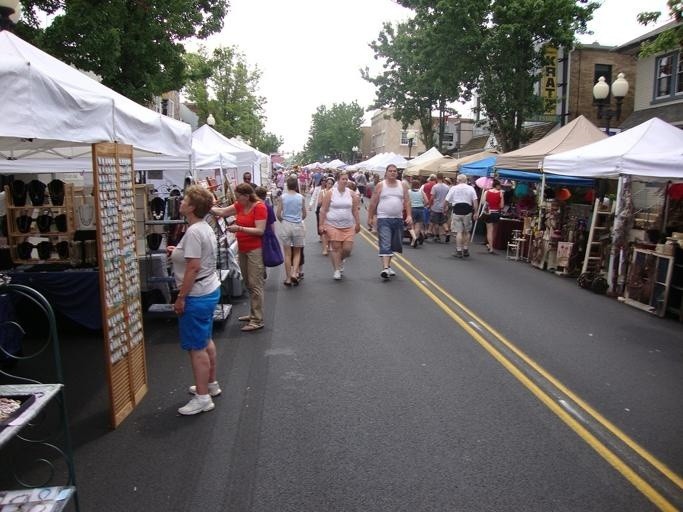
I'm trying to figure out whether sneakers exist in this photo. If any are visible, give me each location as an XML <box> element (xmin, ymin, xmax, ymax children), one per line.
<box><xmin>411</xmin><ymin>231</ymin><xmax>451</xmax><ymax>248</ymax></box>
<box><xmin>463</xmin><ymin>249</ymin><xmax>471</xmax><ymax>257</ymax></box>
<box><xmin>283</xmin><ymin>273</ymin><xmax>305</xmax><ymax>287</ymax></box>
<box><xmin>450</xmin><ymin>250</ymin><xmax>462</xmax><ymax>258</ymax></box>
<box><xmin>187</xmin><ymin>381</ymin><xmax>222</xmax><ymax>397</ymax></box>
<box><xmin>175</xmin><ymin>391</ymin><xmax>215</xmax><ymax>417</ymax></box>
<box><xmin>333</xmin><ymin>266</ymin><xmax>345</xmax><ymax>281</ymax></box>
<box><xmin>379</xmin><ymin>268</ymin><xmax>397</xmax><ymax>280</ymax></box>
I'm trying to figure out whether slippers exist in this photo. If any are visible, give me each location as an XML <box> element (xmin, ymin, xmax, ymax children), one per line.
<box><xmin>239</xmin><ymin>322</ymin><xmax>264</xmax><ymax>332</ymax></box>
<box><xmin>237</xmin><ymin>314</ymin><xmax>249</xmax><ymax>322</ymax></box>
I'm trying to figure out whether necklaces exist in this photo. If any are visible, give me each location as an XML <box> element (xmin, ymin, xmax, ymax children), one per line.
<box><xmin>151</xmin><ymin>198</ymin><xmax>163</xmax><ymax>220</ymax></box>
<box><xmin>9</xmin><ymin>178</ymin><xmax>93</xmax><ymax>261</ymax></box>
<box><xmin>149</xmin><ymin>233</ymin><xmax>159</xmax><ymax>249</ymax></box>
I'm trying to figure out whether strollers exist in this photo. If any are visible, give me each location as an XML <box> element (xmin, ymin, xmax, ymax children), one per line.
<box><xmin>400</xmin><ymin>211</ymin><xmax>426</xmax><ymax>246</ymax></box>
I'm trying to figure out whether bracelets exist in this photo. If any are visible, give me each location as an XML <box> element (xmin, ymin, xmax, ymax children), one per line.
<box><xmin>236</xmin><ymin>225</ymin><xmax>243</xmax><ymax>232</ymax></box>
<box><xmin>177</xmin><ymin>294</ymin><xmax>184</xmax><ymax>298</ymax></box>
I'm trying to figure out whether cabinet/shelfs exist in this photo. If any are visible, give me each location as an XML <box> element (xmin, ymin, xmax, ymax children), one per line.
<box><xmin>494</xmin><ymin>176</ymin><xmax>678</xmax><ymax>320</ymax></box>
<box><xmin>0</xmin><ymin>167</ymin><xmax>193</xmax><ymax>512</ymax></box>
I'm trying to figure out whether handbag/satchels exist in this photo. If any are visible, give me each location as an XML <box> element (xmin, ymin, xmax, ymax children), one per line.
<box><xmin>577</xmin><ymin>271</ymin><xmax>610</xmax><ymax>295</ymax></box>
<box><xmin>261</xmin><ymin>219</ymin><xmax>283</xmax><ymax>266</ymax></box>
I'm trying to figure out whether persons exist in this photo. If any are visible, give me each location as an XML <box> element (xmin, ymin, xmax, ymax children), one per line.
<box><xmin>206</xmin><ymin>182</ymin><xmax>269</xmax><ymax>332</ymax></box>
<box><xmin>274</xmin><ymin>176</ymin><xmax>307</xmax><ymax>286</ymax></box>
<box><xmin>542</xmin><ymin>188</ymin><xmax>561</xmax><ymax>229</ymax></box>
<box><xmin>317</xmin><ymin>171</ymin><xmax>363</xmax><ymax>281</ymax></box>
<box><xmin>254</xmin><ymin>186</ymin><xmax>283</xmax><ymax>279</ymax></box>
<box><xmin>242</xmin><ymin>172</ymin><xmax>258</xmax><ymax>189</ymax></box>
<box><xmin>515</xmin><ymin>187</ymin><xmax>538</xmax><ymax>217</ymax></box>
<box><xmin>164</xmin><ymin>175</ymin><xmax>194</xmax><ymax>248</ymax></box>
<box><xmin>364</xmin><ymin>164</ymin><xmax>413</xmax><ymax>279</ymax></box>
<box><xmin>271</xmin><ymin>162</ymin><xmax>514</xmax><ymax>257</ymax></box>
<box><xmin>163</xmin><ymin>184</ymin><xmax>225</xmax><ymax>417</ymax></box>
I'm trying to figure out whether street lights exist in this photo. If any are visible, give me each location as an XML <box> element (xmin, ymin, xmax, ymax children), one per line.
<box><xmin>592</xmin><ymin>70</ymin><xmax>630</xmax><ymax>136</ymax></box>
<box><xmin>0</xmin><ymin>0</ymin><xmax>25</xmax><ymax>33</ymax></box>
<box><xmin>206</xmin><ymin>113</ymin><xmax>216</xmax><ymax>129</ymax></box>
<box><xmin>405</xmin><ymin>130</ymin><xmax>417</xmax><ymax>159</ymax></box>
<box><xmin>351</xmin><ymin>146</ymin><xmax>359</xmax><ymax>166</ymax></box>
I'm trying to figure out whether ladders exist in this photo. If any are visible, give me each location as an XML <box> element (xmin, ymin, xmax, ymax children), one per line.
<box><xmin>580</xmin><ymin>198</ymin><xmax>617</xmax><ymax>298</ymax></box>
